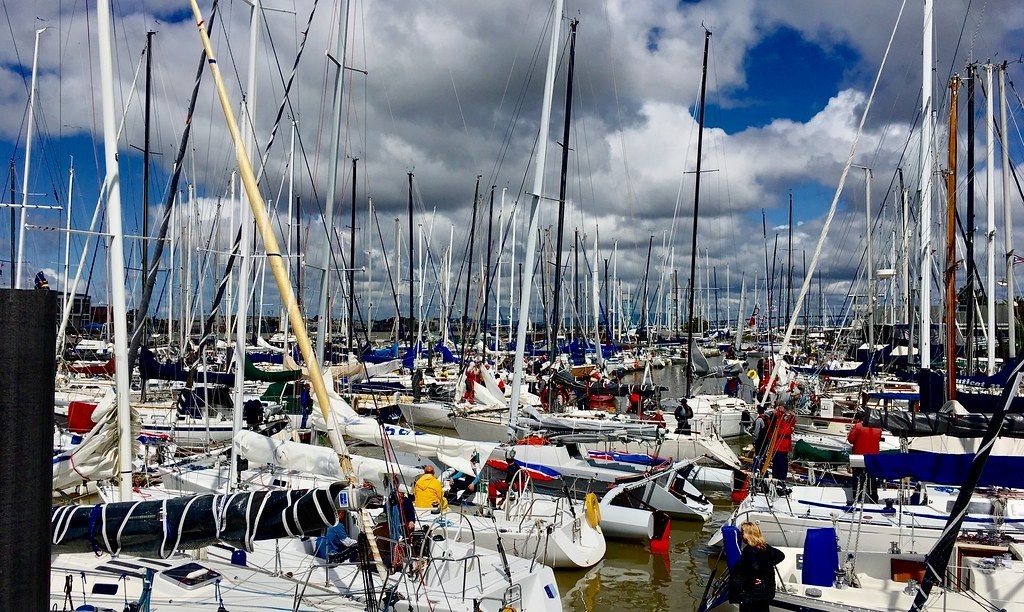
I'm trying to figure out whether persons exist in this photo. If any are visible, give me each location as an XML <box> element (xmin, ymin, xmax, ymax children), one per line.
<box><xmin>727</xmin><ymin>522</ymin><xmax>785</xmax><ymax>612</ymax></box>
<box><xmin>674</xmin><ymin>398</ymin><xmax>693</xmax><ymax>429</ymax></box>
<box><xmin>414</xmin><ymin>465</ymin><xmax>447</xmax><ymax>512</ymax></box>
<box><xmin>724</xmin><ymin>373</ymin><xmax>743</xmax><ymax>397</ymax></box>
<box><xmin>756</xmin><ymin>357</ymin><xmax>773</xmax><ymax>380</ymax></box>
<box><xmin>495</xmin><ymin>374</ymin><xmax>504</xmax><ymax>394</ymax></box>
<box><xmin>412</xmin><ymin>368</ymin><xmax>424</xmax><ymax>403</ymax></box>
<box><xmin>771</xmin><ymin>401</ymin><xmax>795</xmax><ymax>478</ymax></box>
<box><xmin>327</xmin><ymin>510</ymin><xmax>357</xmax><ymax>560</ymax></box>
<box><xmin>527</xmin><ymin>358</ymin><xmax>533</xmax><ymax>368</ymax></box>
<box><xmin>753</xmin><ymin>404</ymin><xmax>771</xmax><ymax>458</ymax></box>
<box><xmin>537</xmin><ymin>375</ymin><xmax>546</xmax><ymax>406</ymax></box>
<box><xmin>300</xmin><ymin>384</ymin><xmax>313</xmax><ymax>429</ymax></box>
<box><xmin>556</xmin><ymin>383</ymin><xmax>570</xmax><ymax>410</ymax></box>
<box><xmin>464</xmin><ymin>362</ymin><xmax>476</xmax><ymax>405</ymax></box>
<box><xmin>488</xmin><ymin>457</ymin><xmax>524</xmax><ymax>507</ymax></box>
<box><xmin>438</xmin><ymin>366</ymin><xmax>448</xmax><ymax>380</ymax></box>
<box><xmin>383</xmin><ymin>491</ymin><xmax>415</xmax><ymax>535</ymax></box>
<box><xmin>449</xmin><ymin>472</ymin><xmax>480</xmax><ymax>503</ymax></box>
<box><xmin>847</xmin><ymin>408</ymin><xmax>882</xmax><ymax>501</ymax></box>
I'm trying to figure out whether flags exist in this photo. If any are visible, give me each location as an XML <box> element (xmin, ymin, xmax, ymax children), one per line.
<box><xmin>1014</xmin><ymin>255</ymin><xmax>1024</xmax><ymax>266</ymax></box>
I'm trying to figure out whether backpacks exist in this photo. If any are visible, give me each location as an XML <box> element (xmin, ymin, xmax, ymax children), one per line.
<box><xmin>685</xmin><ymin>405</ymin><xmax>694</xmax><ymax>418</ymax></box>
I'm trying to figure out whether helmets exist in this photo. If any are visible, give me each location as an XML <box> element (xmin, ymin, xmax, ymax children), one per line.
<box><xmin>424</xmin><ymin>465</ymin><xmax>435</xmax><ymax>473</ymax></box>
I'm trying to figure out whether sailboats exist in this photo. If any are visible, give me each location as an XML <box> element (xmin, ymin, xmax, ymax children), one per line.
<box><xmin>1</xmin><ymin>1</ymin><xmax>1024</xmax><ymax>612</ymax></box>
